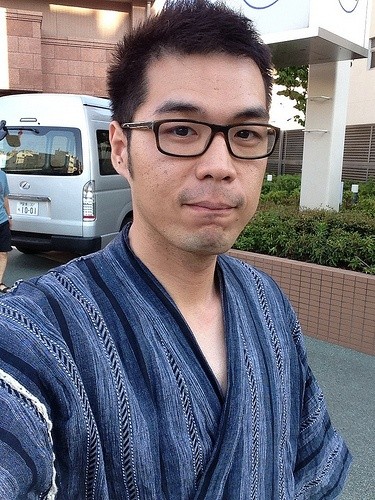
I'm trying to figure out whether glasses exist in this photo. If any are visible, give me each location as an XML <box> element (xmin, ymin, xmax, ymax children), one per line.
<box><xmin>121</xmin><ymin>119</ymin><xmax>280</xmax><ymax>160</ymax></box>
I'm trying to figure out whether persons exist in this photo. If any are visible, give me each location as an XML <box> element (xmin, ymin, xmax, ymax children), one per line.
<box><xmin>0</xmin><ymin>0</ymin><xmax>353</xmax><ymax>500</ymax></box>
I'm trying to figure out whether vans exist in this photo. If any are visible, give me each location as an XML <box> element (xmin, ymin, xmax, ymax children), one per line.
<box><xmin>0</xmin><ymin>94</ymin><xmax>132</xmax><ymax>258</ymax></box>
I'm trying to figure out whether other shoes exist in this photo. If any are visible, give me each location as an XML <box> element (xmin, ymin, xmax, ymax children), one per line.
<box><xmin>0</xmin><ymin>282</ymin><xmax>11</xmax><ymax>293</ymax></box>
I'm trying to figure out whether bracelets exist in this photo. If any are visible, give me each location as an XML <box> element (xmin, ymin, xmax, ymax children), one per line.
<box><xmin>8</xmin><ymin>215</ymin><xmax>12</xmax><ymax>219</ymax></box>
